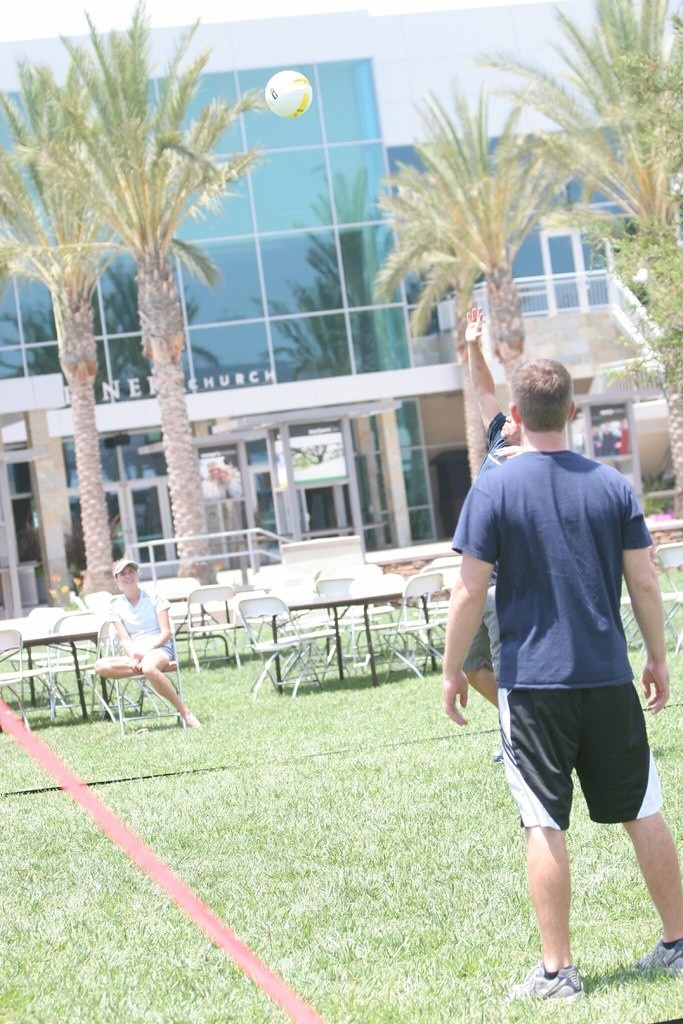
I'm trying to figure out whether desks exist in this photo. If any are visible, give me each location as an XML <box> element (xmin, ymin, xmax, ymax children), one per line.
<box><xmin>22</xmin><ymin>628</ymin><xmax>111</xmax><ymax>723</ymax></box>
<box><xmin>166</xmin><ymin>598</ymin><xmax>232</xmax><ymax>662</ymax></box>
<box><xmin>282</xmin><ymin>591</ymin><xmax>438</xmax><ymax>687</ymax></box>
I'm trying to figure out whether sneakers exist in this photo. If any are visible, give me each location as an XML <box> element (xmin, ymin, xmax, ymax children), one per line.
<box><xmin>507</xmin><ymin>961</ymin><xmax>582</xmax><ymax>1004</ymax></box>
<box><xmin>636</xmin><ymin>938</ymin><xmax>683</xmax><ymax>979</ymax></box>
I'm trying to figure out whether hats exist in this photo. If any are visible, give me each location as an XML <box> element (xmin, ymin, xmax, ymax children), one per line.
<box><xmin>114</xmin><ymin>560</ymin><xmax>139</xmax><ymax>580</ymax></box>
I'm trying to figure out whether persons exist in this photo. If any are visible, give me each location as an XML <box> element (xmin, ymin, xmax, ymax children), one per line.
<box><xmin>592</xmin><ymin>419</ymin><xmax>628</xmax><ymax>456</ymax></box>
<box><xmin>443</xmin><ymin>357</ymin><xmax>683</xmax><ymax>998</ymax></box>
<box><xmin>94</xmin><ymin>558</ymin><xmax>201</xmax><ymax>727</ymax></box>
<box><xmin>201</xmin><ymin>456</ymin><xmax>243</xmax><ymax>500</ymax></box>
<box><xmin>460</xmin><ymin>305</ymin><xmax>523</xmax><ymax>761</ymax></box>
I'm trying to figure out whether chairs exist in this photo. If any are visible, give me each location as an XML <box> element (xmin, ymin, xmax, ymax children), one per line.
<box><xmin>0</xmin><ymin>540</ymin><xmax>683</xmax><ymax>738</ymax></box>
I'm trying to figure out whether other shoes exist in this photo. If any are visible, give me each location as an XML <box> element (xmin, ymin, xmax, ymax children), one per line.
<box><xmin>183</xmin><ymin>712</ymin><xmax>200</xmax><ymax>729</ymax></box>
<box><xmin>493</xmin><ymin>749</ymin><xmax>504</xmax><ymax>764</ymax></box>
<box><xmin>163</xmin><ymin>661</ymin><xmax>178</xmax><ymax>672</ymax></box>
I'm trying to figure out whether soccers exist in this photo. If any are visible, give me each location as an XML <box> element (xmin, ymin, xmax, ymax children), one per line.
<box><xmin>263</xmin><ymin>69</ymin><xmax>315</xmax><ymax>119</ymax></box>
